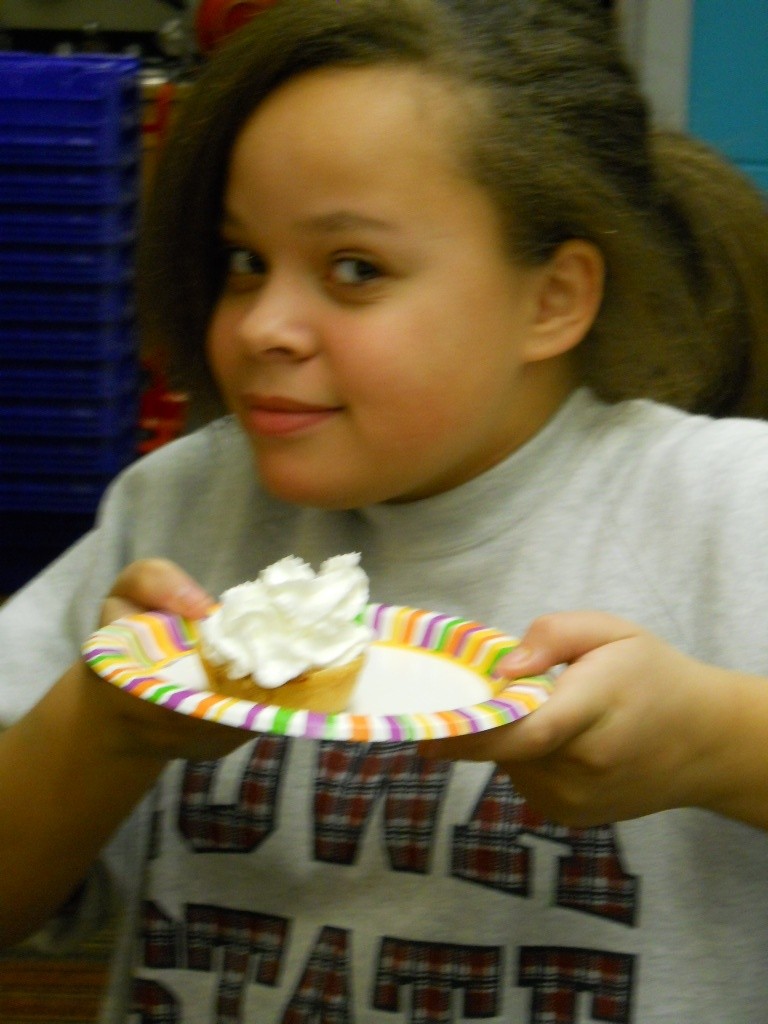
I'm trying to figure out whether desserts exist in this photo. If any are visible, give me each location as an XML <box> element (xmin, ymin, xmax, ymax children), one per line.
<box><xmin>196</xmin><ymin>549</ymin><xmax>370</xmax><ymax>713</ymax></box>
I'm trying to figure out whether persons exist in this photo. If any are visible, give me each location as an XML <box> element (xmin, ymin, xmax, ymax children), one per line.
<box><xmin>0</xmin><ymin>0</ymin><xmax>767</xmax><ymax>1024</ymax></box>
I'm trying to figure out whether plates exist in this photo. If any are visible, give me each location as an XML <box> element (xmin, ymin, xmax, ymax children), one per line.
<box><xmin>80</xmin><ymin>602</ymin><xmax>560</xmax><ymax>743</ymax></box>
